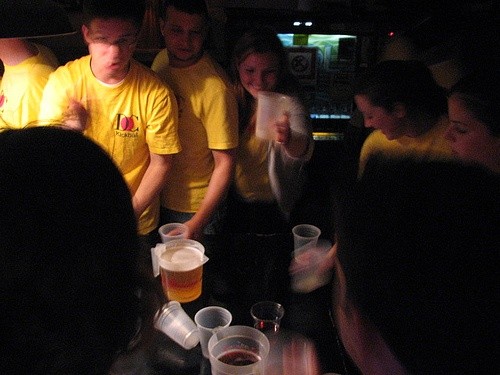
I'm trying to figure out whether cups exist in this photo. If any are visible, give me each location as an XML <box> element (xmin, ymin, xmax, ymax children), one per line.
<box><xmin>157</xmin><ymin>223</ymin><xmax>189</xmax><ymax>242</ymax></box>
<box><xmin>154</xmin><ymin>239</ymin><xmax>210</xmax><ymax>302</ymax></box>
<box><xmin>250</xmin><ymin>300</ymin><xmax>284</xmax><ymax>335</ymax></box>
<box><xmin>292</xmin><ymin>224</ymin><xmax>322</xmax><ymax>263</ymax></box>
<box><xmin>152</xmin><ymin>300</ymin><xmax>200</xmax><ymax>350</ymax></box>
<box><xmin>256</xmin><ymin>91</ymin><xmax>289</xmax><ymax>139</ymax></box>
<box><xmin>194</xmin><ymin>306</ymin><xmax>233</xmax><ymax>358</ymax></box>
<box><xmin>208</xmin><ymin>325</ymin><xmax>270</xmax><ymax>375</ymax></box>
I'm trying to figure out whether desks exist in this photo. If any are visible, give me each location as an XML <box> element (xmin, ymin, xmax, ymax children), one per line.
<box><xmin>151</xmin><ymin>234</ymin><xmax>347</xmax><ymax>375</ymax></box>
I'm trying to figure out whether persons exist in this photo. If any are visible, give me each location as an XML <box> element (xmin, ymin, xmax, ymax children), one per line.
<box><xmin>262</xmin><ymin>149</ymin><xmax>500</xmax><ymax>375</ymax></box>
<box><xmin>355</xmin><ymin>58</ymin><xmax>455</xmax><ymax>181</ymax></box>
<box><xmin>0</xmin><ymin>124</ymin><xmax>169</xmax><ymax>375</ymax></box>
<box><xmin>151</xmin><ymin>0</ymin><xmax>238</xmax><ymax>255</ymax></box>
<box><xmin>0</xmin><ymin>0</ymin><xmax>79</xmax><ymax>132</ymax></box>
<box><xmin>37</xmin><ymin>0</ymin><xmax>182</xmax><ymax>249</ymax></box>
<box><xmin>446</xmin><ymin>70</ymin><xmax>499</xmax><ymax>174</ymax></box>
<box><xmin>230</xmin><ymin>24</ymin><xmax>314</xmax><ymax>236</ymax></box>
